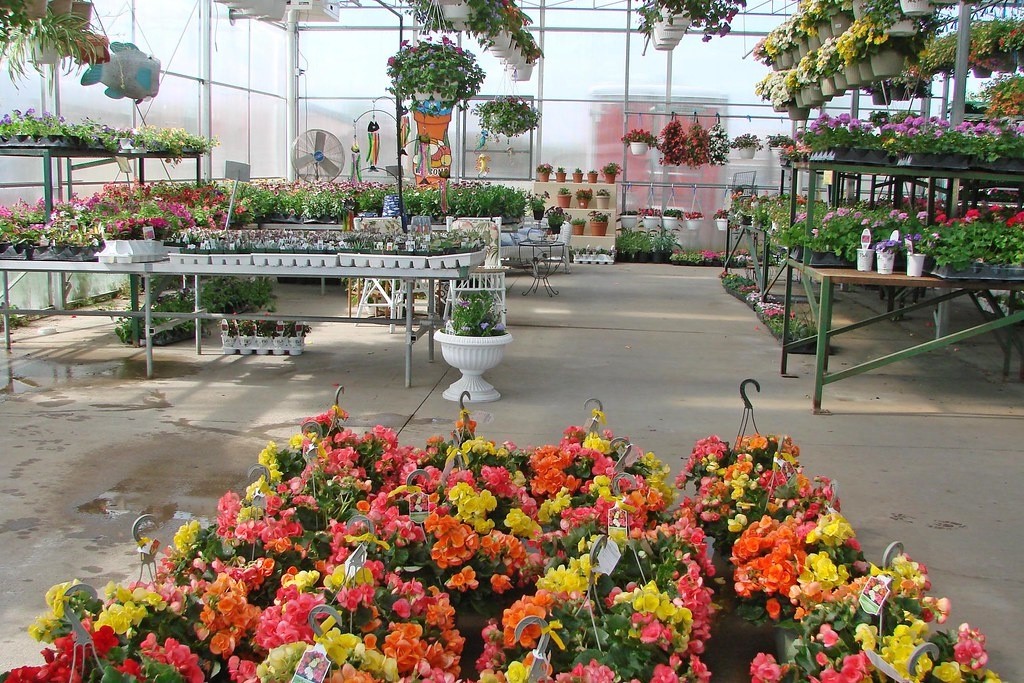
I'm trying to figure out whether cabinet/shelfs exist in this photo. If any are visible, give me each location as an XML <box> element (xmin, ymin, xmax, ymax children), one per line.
<box><xmin>532</xmin><ymin>181</ymin><xmax>617</xmax><ymax>253</ymax></box>
<box><xmin>780</xmin><ymin>162</ymin><xmax>1024</xmax><ymax>415</ymax></box>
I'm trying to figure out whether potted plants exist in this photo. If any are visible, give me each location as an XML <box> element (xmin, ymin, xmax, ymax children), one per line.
<box><xmin>620</xmin><ymin>227</ymin><xmax>640</xmax><ymax>263</ymax></box>
<box><xmin>636</xmin><ymin>228</ymin><xmax>653</xmax><ymax>263</ymax></box>
<box><xmin>0</xmin><ymin>10</ymin><xmax>102</xmax><ymax>91</ymax></box>
<box><xmin>614</xmin><ymin>234</ymin><xmax>630</xmax><ymax>263</ymax></box>
<box><xmin>648</xmin><ymin>224</ymin><xmax>665</xmax><ymax>264</ymax></box>
<box><xmin>660</xmin><ymin>230</ymin><xmax>683</xmax><ymax>264</ymax></box>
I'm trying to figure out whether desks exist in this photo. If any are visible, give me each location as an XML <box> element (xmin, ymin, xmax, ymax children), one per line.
<box><xmin>0</xmin><ymin>260</ymin><xmax>486</xmax><ymax>388</ymax></box>
<box><xmin>259</xmin><ymin>224</ymin><xmax>343</xmax><ymax>295</ymax></box>
<box><xmin>517</xmin><ymin>241</ymin><xmax>565</xmax><ymax>297</ymax></box>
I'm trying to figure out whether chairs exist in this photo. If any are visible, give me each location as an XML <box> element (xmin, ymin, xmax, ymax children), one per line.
<box><xmin>354</xmin><ymin>218</ymin><xmax>436</xmax><ymax>335</ymax></box>
<box><xmin>443</xmin><ymin>217</ymin><xmax>507</xmax><ymax>328</ymax></box>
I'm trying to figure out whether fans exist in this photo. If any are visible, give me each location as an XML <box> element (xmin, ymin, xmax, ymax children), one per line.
<box><xmin>290</xmin><ymin>129</ymin><xmax>345</xmax><ymax>183</ymax></box>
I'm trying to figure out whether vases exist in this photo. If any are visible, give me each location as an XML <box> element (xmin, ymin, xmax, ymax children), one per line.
<box><xmin>662</xmin><ymin>216</ymin><xmax>680</xmax><ymax>230</ymax></box>
<box><xmin>587</xmin><ymin>173</ymin><xmax>598</xmax><ymax>183</ymax></box>
<box><xmin>578</xmin><ymin>198</ymin><xmax>589</xmax><ymax>209</ymax></box>
<box><xmin>641</xmin><ymin>216</ymin><xmax>659</xmax><ymax>229</ymax></box>
<box><xmin>769</xmin><ymin>147</ymin><xmax>783</xmax><ymax>161</ymax></box>
<box><xmin>573</xmin><ymin>174</ymin><xmax>583</xmax><ymax>183</ymax></box>
<box><xmin>603</xmin><ymin>173</ymin><xmax>616</xmax><ymax>184</ymax></box>
<box><xmin>685</xmin><ymin>217</ymin><xmax>704</xmax><ymax>230</ymax></box>
<box><xmin>22</xmin><ymin>0</ymin><xmax>48</xmax><ymax>19</ymax></box>
<box><xmin>442</xmin><ymin>2</ymin><xmax>471</xmax><ymax>19</ymax></box>
<box><xmin>972</xmin><ymin>65</ymin><xmax>992</xmax><ymax>78</ymax></box>
<box><xmin>413</xmin><ymin>81</ymin><xmax>457</xmax><ymax>101</ymax></box>
<box><xmin>433</xmin><ymin>328</ymin><xmax>513</xmax><ymax>403</ymax></box>
<box><xmin>629</xmin><ymin>142</ymin><xmax>649</xmax><ymax>156</ymax></box>
<box><xmin>739</xmin><ymin>147</ymin><xmax>755</xmax><ymax>160</ymax></box>
<box><xmin>556</xmin><ymin>172</ymin><xmax>566</xmax><ymax>182</ymax></box>
<box><xmin>71</xmin><ymin>3</ymin><xmax>94</xmax><ymax>30</ymax></box>
<box><xmin>620</xmin><ymin>215</ymin><xmax>637</xmax><ymax>228</ymax></box>
<box><xmin>856</xmin><ymin>249</ymin><xmax>875</xmax><ymax>272</ymax></box>
<box><xmin>715</xmin><ymin>218</ymin><xmax>727</xmax><ymax>231</ymax></box>
<box><xmin>907</xmin><ymin>253</ymin><xmax>926</xmax><ymax>277</ymax></box>
<box><xmin>899</xmin><ymin>0</ymin><xmax>935</xmax><ymax>17</ymax></box>
<box><xmin>871</xmin><ymin>90</ymin><xmax>891</xmax><ymax>106</ymax></box>
<box><xmin>595</xmin><ymin>196</ymin><xmax>610</xmax><ymax>209</ymax></box>
<box><xmin>590</xmin><ymin>222</ymin><xmax>608</xmax><ymax>236</ymax></box>
<box><xmin>538</xmin><ymin>172</ymin><xmax>550</xmax><ymax>182</ymax></box>
<box><xmin>884</xmin><ymin>17</ymin><xmax>919</xmax><ymax>36</ymax></box>
<box><xmin>533</xmin><ymin>210</ymin><xmax>544</xmax><ymax>220</ymax></box>
<box><xmin>876</xmin><ymin>251</ymin><xmax>895</xmax><ymax>274</ymax></box>
<box><xmin>870</xmin><ymin>49</ymin><xmax>905</xmax><ymax>77</ymax></box>
<box><xmin>557</xmin><ymin>195</ymin><xmax>571</xmax><ymax>208</ymax></box>
<box><xmin>508</xmin><ymin>64</ymin><xmax>532</xmax><ymax>81</ymax></box>
<box><xmin>550</xmin><ymin>224</ymin><xmax>561</xmax><ymax>234</ymax></box>
<box><xmin>47</xmin><ymin>0</ymin><xmax>73</xmax><ymax>26</ymax></box>
<box><xmin>572</xmin><ymin>224</ymin><xmax>584</xmax><ymax>236</ymax></box>
<box><xmin>890</xmin><ymin>85</ymin><xmax>910</xmax><ymax>102</ymax></box>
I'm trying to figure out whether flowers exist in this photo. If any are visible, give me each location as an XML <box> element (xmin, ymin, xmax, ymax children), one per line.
<box><xmin>0</xmin><ymin>0</ymin><xmax>1024</xmax><ymax>683</ymax></box>
